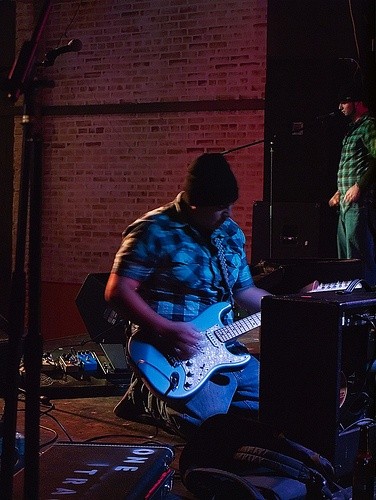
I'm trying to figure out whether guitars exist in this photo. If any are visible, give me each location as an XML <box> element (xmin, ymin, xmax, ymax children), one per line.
<box><xmin>128</xmin><ymin>278</ymin><xmax>363</xmax><ymax>400</ymax></box>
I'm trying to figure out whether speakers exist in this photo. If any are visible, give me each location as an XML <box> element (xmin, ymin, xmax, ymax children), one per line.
<box><xmin>76</xmin><ymin>272</ymin><xmax>131</xmax><ymax>343</ymax></box>
<box><xmin>259</xmin><ymin>285</ymin><xmax>376</xmax><ymax>432</ymax></box>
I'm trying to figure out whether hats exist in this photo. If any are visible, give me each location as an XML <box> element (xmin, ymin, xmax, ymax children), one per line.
<box><xmin>182</xmin><ymin>153</ymin><xmax>239</xmax><ymax>208</ymax></box>
<box><xmin>335</xmin><ymin>83</ymin><xmax>363</xmax><ymax>101</ymax></box>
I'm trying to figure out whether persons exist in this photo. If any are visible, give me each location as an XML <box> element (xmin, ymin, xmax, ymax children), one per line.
<box><xmin>104</xmin><ymin>151</ymin><xmax>278</xmax><ymax>432</ymax></box>
<box><xmin>328</xmin><ymin>81</ymin><xmax>376</xmax><ymax>286</ymax></box>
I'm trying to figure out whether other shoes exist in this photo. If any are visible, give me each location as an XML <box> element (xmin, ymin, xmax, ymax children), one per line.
<box><xmin>113</xmin><ymin>377</ymin><xmax>143</xmax><ymax>417</ymax></box>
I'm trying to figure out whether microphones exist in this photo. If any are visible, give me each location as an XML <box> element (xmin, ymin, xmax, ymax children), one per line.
<box><xmin>316</xmin><ymin>112</ymin><xmax>336</xmax><ymax>120</ymax></box>
<box><xmin>44</xmin><ymin>39</ymin><xmax>82</xmax><ymax>58</ymax></box>
